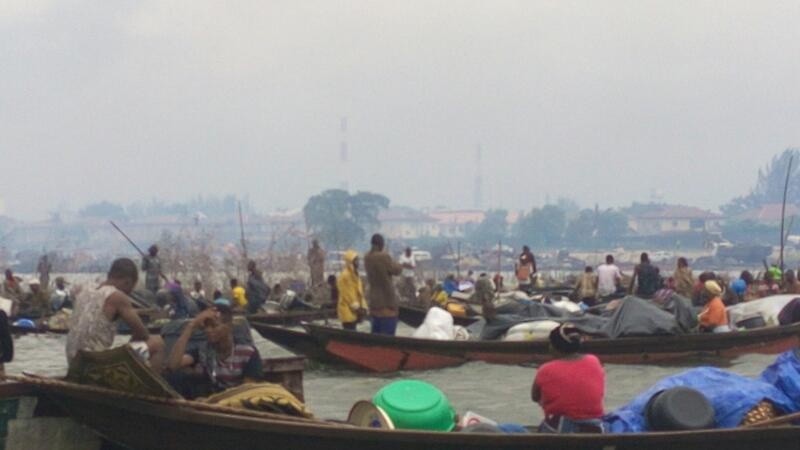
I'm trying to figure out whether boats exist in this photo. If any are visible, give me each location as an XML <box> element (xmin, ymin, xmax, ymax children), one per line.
<box><xmin>23</xmin><ymin>340</ymin><xmax>800</xmax><ymax>450</ymax></box>
<box><xmin>3</xmin><ymin>261</ymin><xmax>800</xmax><ymax>340</ymax></box>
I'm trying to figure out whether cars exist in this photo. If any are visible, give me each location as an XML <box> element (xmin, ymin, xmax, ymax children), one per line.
<box><xmin>491</xmin><ymin>242</ymin><xmax>516</xmax><ymax>258</ymax></box>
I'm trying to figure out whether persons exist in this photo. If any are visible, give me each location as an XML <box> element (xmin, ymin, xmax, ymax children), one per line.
<box><xmin>65</xmin><ymin>258</ymin><xmax>163</xmax><ymax>378</ymax></box>
<box><xmin>364</xmin><ymin>234</ymin><xmax>402</xmax><ymax>337</ymax></box>
<box><xmin>243</xmin><ymin>261</ymin><xmax>271</xmax><ymax>314</ymax></box>
<box><xmin>575</xmin><ymin>266</ymin><xmax>599</xmax><ymax>306</ymax></box>
<box><xmin>166</xmin><ymin>278</ymin><xmax>284</xmax><ymax>315</ymax></box>
<box><xmin>170</xmin><ymin>303</ymin><xmax>263</xmax><ymax>400</ymax></box>
<box><xmin>308</xmin><ymin>239</ymin><xmax>326</xmax><ymax>288</ymax></box>
<box><xmin>336</xmin><ymin>250</ymin><xmax>368</xmax><ymax>330</ymax></box>
<box><xmin>597</xmin><ymin>255</ymin><xmax>627</xmax><ymax>302</ymax></box>
<box><xmin>697</xmin><ymin>280</ymin><xmax>728</xmax><ymax>331</ymax></box>
<box><xmin>0</xmin><ymin>268</ymin><xmax>73</xmax><ymax>321</ymax></box>
<box><xmin>531</xmin><ymin>323</ymin><xmax>606</xmax><ymax>435</ymax></box>
<box><xmin>142</xmin><ymin>246</ymin><xmax>162</xmax><ymax>295</ymax></box>
<box><xmin>629</xmin><ymin>253</ymin><xmax>800</xmax><ymax>308</ymax></box>
<box><xmin>515</xmin><ymin>245</ymin><xmax>537</xmax><ymax>293</ymax></box>
<box><xmin>398</xmin><ymin>247</ymin><xmax>417</xmax><ymax>287</ymax></box>
<box><xmin>36</xmin><ymin>254</ymin><xmax>51</xmax><ymax>290</ymax></box>
<box><xmin>279</xmin><ymin>270</ymin><xmax>506</xmax><ymax>312</ymax></box>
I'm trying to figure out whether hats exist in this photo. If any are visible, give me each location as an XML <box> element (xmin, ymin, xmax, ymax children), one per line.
<box><xmin>704</xmin><ymin>279</ymin><xmax>722</xmax><ymax>296</ymax></box>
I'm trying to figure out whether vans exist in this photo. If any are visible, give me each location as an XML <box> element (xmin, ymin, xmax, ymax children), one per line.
<box><xmin>400</xmin><ymin>251</ymin><xmax>432</xmax><ymax>262</ymax></box>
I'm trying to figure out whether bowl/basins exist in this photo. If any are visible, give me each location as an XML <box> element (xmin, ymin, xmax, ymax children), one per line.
<box><xmin>347</xmin><ymin>380</ymin><xmax>456</xmax><ymax>432</ymax></box>
